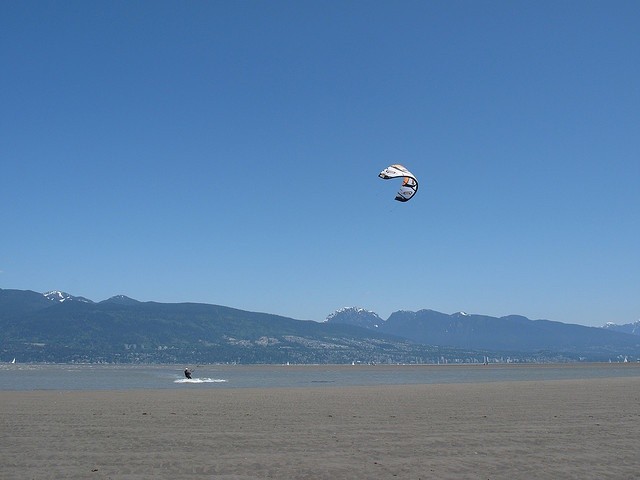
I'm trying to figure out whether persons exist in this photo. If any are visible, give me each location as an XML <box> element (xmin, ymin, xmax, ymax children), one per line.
<box><xmin>184</xmin><ymin>368</ymin><xmax>193</xmax><ymax>379</ymax></box>
